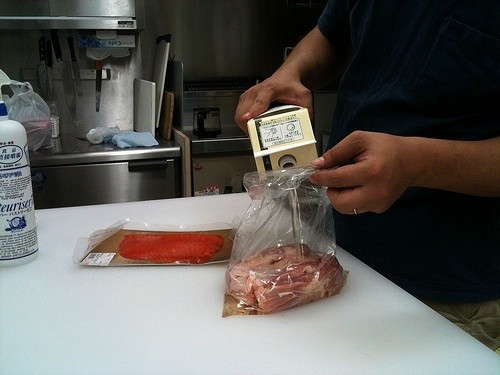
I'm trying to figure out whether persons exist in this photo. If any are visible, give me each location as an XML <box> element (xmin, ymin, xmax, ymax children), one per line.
<box><xmin>234</xmin><ymin>0</ymin><xmax>500</xmax><ymax>353</ymax></box>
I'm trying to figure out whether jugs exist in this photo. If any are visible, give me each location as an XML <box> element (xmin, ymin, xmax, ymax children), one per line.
<box><xmin>192</xmin><ymin>108</ymin><xmax>222</xmax><ymax>136</ymax></box>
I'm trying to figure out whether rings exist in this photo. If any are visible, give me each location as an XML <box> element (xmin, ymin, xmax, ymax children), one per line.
<box><xmin>353</xmin><ymin>209</ymin><xmax>358</xmax><ymax>215</ymax></box>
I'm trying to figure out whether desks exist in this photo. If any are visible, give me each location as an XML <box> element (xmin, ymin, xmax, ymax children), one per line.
<box><xmin>0</xmin><ymin>190</ymin><xmax>500</xmax><ymax>375</ymax></box>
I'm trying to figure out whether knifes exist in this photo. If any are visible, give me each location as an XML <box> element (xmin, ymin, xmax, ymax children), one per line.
<box><xmin>38</xmin><ymin>29</ymin><xmax>82</xmax><ymax>97</ymax></box>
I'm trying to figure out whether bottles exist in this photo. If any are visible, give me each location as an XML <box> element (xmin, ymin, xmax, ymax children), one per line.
<box><xmin>0</xmin><ymin>101</ymin><xmax>39</xmax><ymax>267</ymax></box>
